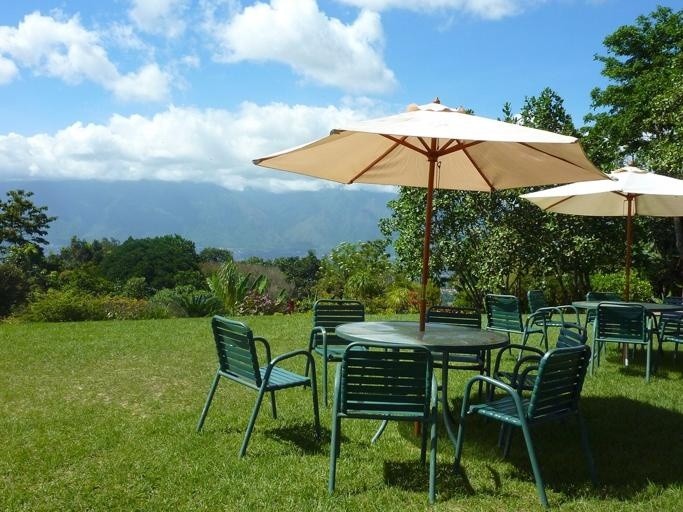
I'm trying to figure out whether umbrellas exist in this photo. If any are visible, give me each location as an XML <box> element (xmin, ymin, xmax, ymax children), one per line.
<box><xmin>248</xmin><ymin>95</ymin><xmax>620</xmax><ymax>334</ymax></box>
<box><xmin>516</xmin><ymin>162</ymin><xmax>683</xmax><ymax>303</ymax></box>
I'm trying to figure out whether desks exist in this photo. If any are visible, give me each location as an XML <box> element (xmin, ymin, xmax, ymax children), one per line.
<box><xmin>333</xmin><ymin>318</ymin><xmax>506</xmax><ymax>443</ymax></box>
<box><xmin>571</xmin><ymin>299</ymin><xmax>681</xmax><ymax>362</ymax></box>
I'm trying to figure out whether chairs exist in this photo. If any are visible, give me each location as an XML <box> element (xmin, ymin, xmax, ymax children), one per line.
<box><xmin>196</xmin><ymin>315</ymin><xmax>323</xmax><ymax>462</ymax></box>
<box><xmin>425</xmin><ymin>306</ymin><xmax>485</xmax><ymax>395</ymax></box>
<box><xmin>527</xmin><ymin>291</ymin><xmax>577</xmax><ymax>345</ymax></box>
<box><xmin>304</xmin><ymin>299</ymin><xmax>372</xmax><ymax>401</ymax></box>
<box><xmin>590</xmin><ymin>300</ymin><xmax>652</xmax><ymax>384</ymax></box>
<box><xmin>653</xmin><ymin>297</ymin><xmax>681</xmax><ymax>362</ymax></box>
<box><xmin>454</xmin><ymin>345</ymin><xmax>593</xmax><ymax>506</ymax></box>
<box><xmin>489</xmin><ymin>324</ymin><xmax>587</xmax><ymax>400</ymax></box>
<box><xmin>486</xmin><ymin>293</ymin><xmax>546</xmax><ymax>364</ymax></box>
<box><xmin>327</xmin><ymin>342</ymin><xmax>438</xmax><ymax>505</ymax></box>
